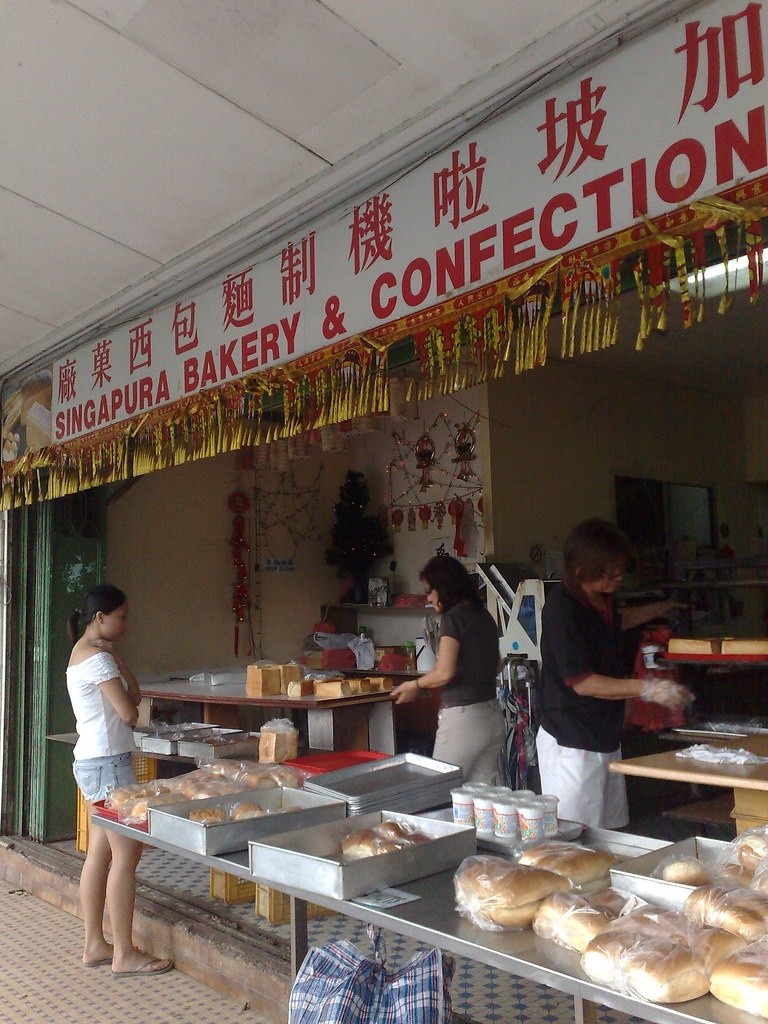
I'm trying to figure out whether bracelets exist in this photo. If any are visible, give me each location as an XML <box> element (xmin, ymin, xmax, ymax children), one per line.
<box><xmin>415</xmin><ymin>679</ymin><xmax>430</xmax><ymax>697</ymax></box>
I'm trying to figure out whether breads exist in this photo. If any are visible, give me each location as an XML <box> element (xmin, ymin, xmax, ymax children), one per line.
<box><xmin>340</xmin><ymin>821</ymin><xmax>433</xmax><ymax>858</ymax></box>
<box><xmin>456</xmin><ymin>830</ymin><xmax>768</xmax><ymax>1019</ymax></box>
<box><xmin>259</xmin><ymin>728</ymin><xmax>299</xmax><ymax>763</ymax></box>
<box><xmin>106</xmin><ymin>756</ymin><xmax>308</xmax><ymax>824</ymax></box>
<box><xmin>668</xmin><ymin>634</ymin><xmax>768</xmax><ymax>655</ymax></box>
<box><xmin>246</xmin><ymin>663</ymin><xmax>392</xmax><ymax>697</ymax></box>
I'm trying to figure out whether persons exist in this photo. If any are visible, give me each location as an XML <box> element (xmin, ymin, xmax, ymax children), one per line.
<box><xmin>535</xmin><ymin>517</ymin><xmax>697</xmax><ymax>830</ymax></box>
<box><xmin>65</xmin><ymin>584</ymin><xmax>172</xmax><ymax>978</ymax></box>
<box><xmin>389</xmin><ymin>555</ymin><xmax>506</xmax><ymax>785</ymax></box>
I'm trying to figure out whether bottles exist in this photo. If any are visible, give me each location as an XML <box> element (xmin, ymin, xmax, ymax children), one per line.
<box><xmin>355</xmin><ymin>627</ymin><xmax>369</xmax><ymax>667</ymax></box>
<box><xmin>402</xmin><ymin>641</ymin><xmax>418</xmax><ymax>673</ymax></box>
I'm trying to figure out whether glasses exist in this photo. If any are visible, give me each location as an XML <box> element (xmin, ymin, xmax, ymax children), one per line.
<box><xmin>425</xmin><ymin>586</ymin><xmax>436</xmax><ymax>596</ymax></box>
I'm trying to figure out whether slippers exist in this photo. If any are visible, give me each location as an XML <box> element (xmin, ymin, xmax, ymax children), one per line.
<box><xmin>84</xmin><ymin>946</ymin><xmax>113</xmax><ymax>967</ymax></box>
<box><xmin>110</xmin><ymin>955</ymin><xmax>173</xmax><ymax>978</ymax></box>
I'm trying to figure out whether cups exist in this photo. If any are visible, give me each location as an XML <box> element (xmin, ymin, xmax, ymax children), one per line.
<box><xmin>516</xmin><ymin>801</ymin><xmax>543</xmax><ymax>842</ymax></box>
<box><xmin>462</xmin><ymin>782</ymin><xmax>486</xmax><ymax>794</ymax></box>
<box><xmin>485</xmin><ymin>785</ymin><xmax>511</xmax><ymax>798</ymax></box>
<box><xmin>534</xmin><ymin>794</ymin><xmax>559</xmax><ymax>837</ymax></box>
<box><xmin>473</xmin><ymin>793</ymin><xmax>495</xmax><ymax>833</ymax></box>
<box><xmin>510</xmin><ymin>789</ymin><xmax>535</xmax><ymax>804</ymax></box>
<box><xmin>492</xmin><ymin>796</ymin><xmax>517</xmax><ymax>839</ymax></box>
<box><xmin>451</xmin><ymin>787</ymin><xmax>475</xmax><ymax>828</ymax></box>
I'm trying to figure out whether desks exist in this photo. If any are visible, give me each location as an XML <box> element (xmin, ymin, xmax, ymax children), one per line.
<box><xmin>94</xmin><ymin>749</ymin><xmax>768</xmax><ymax>1024</ymax></box>
<box><xmin>134</xmin><ymin>677</ymin><xmax>400</xmax><ymax>757</ymax></box>
<box><xmin>609</xmin><ymin>733</ymin><xmax>768</xmax><ymax>835</ymax></box>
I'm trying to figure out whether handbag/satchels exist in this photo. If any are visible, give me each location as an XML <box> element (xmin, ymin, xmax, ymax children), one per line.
<box><xmin>287</xmin><ymin>926</ymin><xmax>456</xmax><ymax>1024</ymax></box>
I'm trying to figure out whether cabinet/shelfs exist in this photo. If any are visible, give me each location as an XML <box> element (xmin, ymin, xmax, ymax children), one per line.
<box><xmin>652</xmin><ymin>656</ymin><xmax>768</xmax><ymax>828</ymax></box>
<box><xmin>321</xmin><ymin>602</ymin><xmax>443</xmax><ymax>676</ymax></box>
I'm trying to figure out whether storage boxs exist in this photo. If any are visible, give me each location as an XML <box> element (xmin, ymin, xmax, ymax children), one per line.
<box><xmin>254</xmin><ymin>882</ymin><xmax>339</xmax><ymax>927</ymax></box>
<box><xmin>76</xmin><ymin>754</ymin><xmax>157</xmax><ymax>854</ymax></box>
<box><xmin>210</xmin><ymin>866</ymin><xmax>256</xmax><ymax>904</ymax></box>
